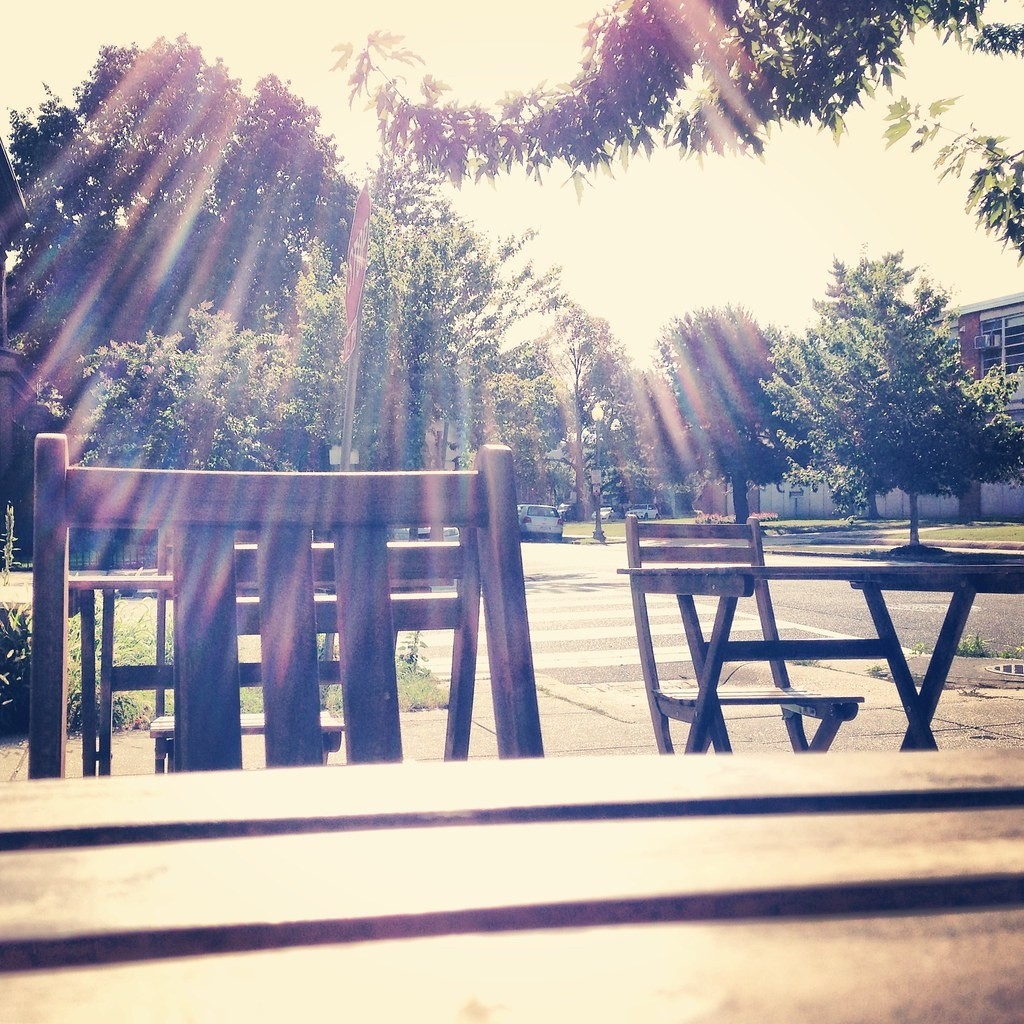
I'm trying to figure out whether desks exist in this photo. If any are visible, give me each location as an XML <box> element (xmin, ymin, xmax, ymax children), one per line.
<box><xmin>0</xmin><ymin>753</ymin><xmax>1023</xmax><ymax>1023</ymax></box>
<box><xmin>613</xmin><ymin>557</ymin><xmax>1024</xmax><ymax>755</ymax></box>
<box><xmin>63</xmin><ymin>575</ymin><xmax>454</xmax><ymax>775</ymax></box>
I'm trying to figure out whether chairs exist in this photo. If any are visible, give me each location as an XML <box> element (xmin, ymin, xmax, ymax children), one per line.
<box><xmin>626</xmin><ymin>514</ymin><xmax>864</xmax><ymax>755</ymax></box>
<box><xmin>27</xmin><ymin>433</ymin><xmax>545</xmax><ymax>779</ymax></box>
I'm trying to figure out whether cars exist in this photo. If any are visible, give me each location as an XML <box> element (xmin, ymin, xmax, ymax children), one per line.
<box><xmin>625</xmin><ymin>503</ymin><xmax>661</xmax><ymax>520</ymax></box>
<box><xmin>556</xmin><ymin>503</ymin><xmax>574</xmax><ymax>521</ymax></box>
<box><xmin>591</xmin><ymin>507</ymin><xmax>624</xmax><ymax>521</ymax></box>
<box><xmin>517</xmin><ymin>504</ymin><xmax>564</xmax><ymax>540</ymax></box>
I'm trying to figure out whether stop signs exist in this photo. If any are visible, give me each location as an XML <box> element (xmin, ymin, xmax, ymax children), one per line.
<box><xmin>345</xmin><ymin>182</ymin><xmax>371</xmax><ymax>331</ymax></box>
